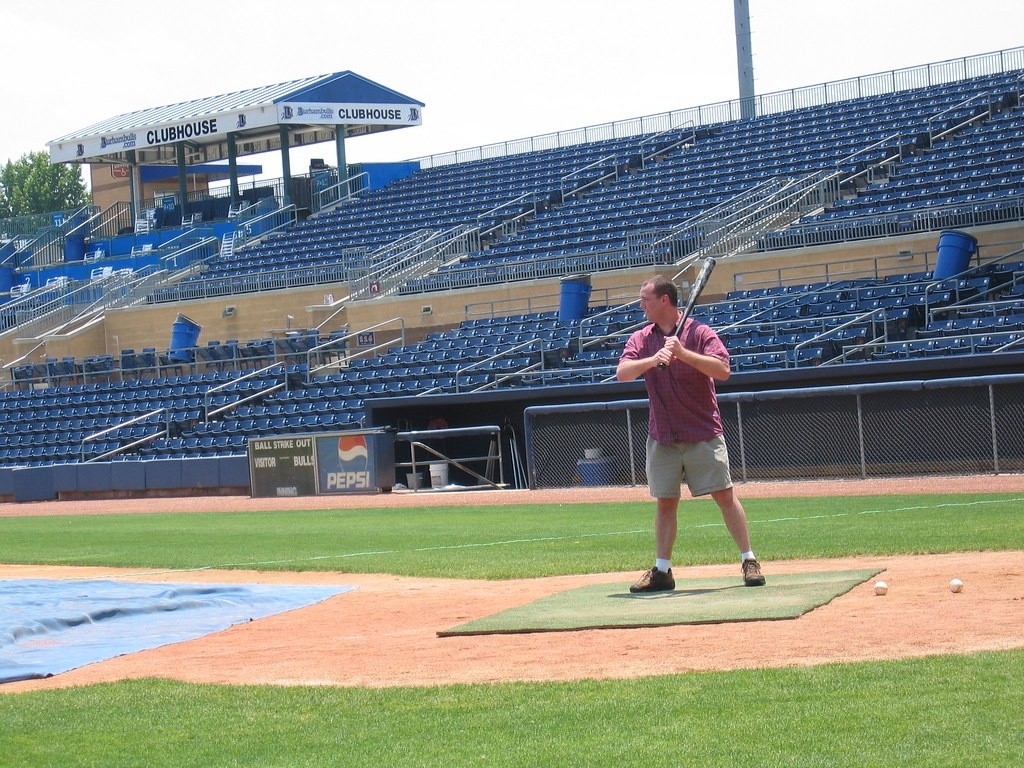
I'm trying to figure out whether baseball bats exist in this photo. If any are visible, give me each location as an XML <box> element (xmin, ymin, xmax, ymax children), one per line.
<box><xmin>657</xmin><ymin>257</ymin><xmax>717</xmax><ymax>371</ymax></box>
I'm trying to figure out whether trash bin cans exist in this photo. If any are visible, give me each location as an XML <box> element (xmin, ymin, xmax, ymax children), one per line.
<box><xmin>559</xmin><ymin>273</ymin><xmax>593</xmax><ymax>322</ymax></box>
<box><xmin>168</xmin><ymin>312</ymin><xmax>204</xmax><ymax>363</ymax></box>
<box><xmin>932</xmin><ymin>228</ymin><xmax>978</xmax><ymax>281</ymax></box>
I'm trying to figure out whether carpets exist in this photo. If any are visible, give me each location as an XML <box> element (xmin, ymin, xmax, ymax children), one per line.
<box><xmin>0</xmin><ymin>579</ymin><xmax>357</xmax><ymax>690</ymax></box>
<box><xmin>435</xmin><ymin>567</ymin><xmax>887</xmax><ymax>639</ymax></box>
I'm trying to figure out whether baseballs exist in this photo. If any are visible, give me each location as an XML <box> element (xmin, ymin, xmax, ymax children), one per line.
<box><xmin>949</xmin><ymin>578</ymin><xmax>964</xmax><ymax>593</ymax></box>
<box><xmin>874</xmin><ymin>581</ymin><xmax>888</xmax><ymax>595</ymax></box>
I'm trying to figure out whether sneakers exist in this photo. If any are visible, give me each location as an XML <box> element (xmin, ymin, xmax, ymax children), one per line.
<box><xmin>740</xmin><ymin>559</ymin><xmax>765</xmax><ymax>584</ymax></box>
<box><xmin>630</xmin><ymin>566</ymin><xmax>675</xmax><ymax>593</ymax></box>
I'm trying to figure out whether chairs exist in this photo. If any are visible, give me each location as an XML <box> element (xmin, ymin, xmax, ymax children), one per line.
<box><xmin>0</xmin><ymin>107</ymin><xmax>1024</xmax><ymax>469</ymax></box>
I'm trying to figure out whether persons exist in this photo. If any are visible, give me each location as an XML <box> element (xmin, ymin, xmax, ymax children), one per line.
<box><xmin>616</xmin><ymin>274</ymin><xmax>766</xmax><ymax>593</ymax></box>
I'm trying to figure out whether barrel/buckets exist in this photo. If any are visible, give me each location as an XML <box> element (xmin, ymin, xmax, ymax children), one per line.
<box><xmin>168</xmin><ymin>313</ymin><xmax>203</xmax><ymax>363</ymax></box>
<box><xmin>559</xmin><ymin>272</ymin><xmax>593</xmax><ymax>322</ymax></box>
<box><xmin>932</xmin><ymin>229</ymin><xmax>978</xmax><ymax>280</ymax></box>
<box><xmin>429</xmin><ymin>464</ymin><xmax>449</xmax><ymax>488</ymax></box>
<box><xmin>406</xmin><ymin>473</ymin><xmax>423</xmax><ymax>488</ymax></box>
